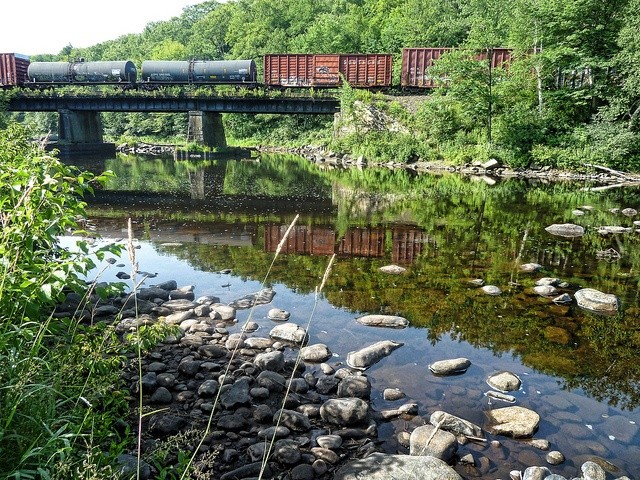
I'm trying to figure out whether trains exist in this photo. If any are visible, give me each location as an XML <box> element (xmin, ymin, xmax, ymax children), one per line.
<box><xmin>1</xmin><ymin>45</ymin><xmax>592</xmax><ymax>89</ymax></box>
